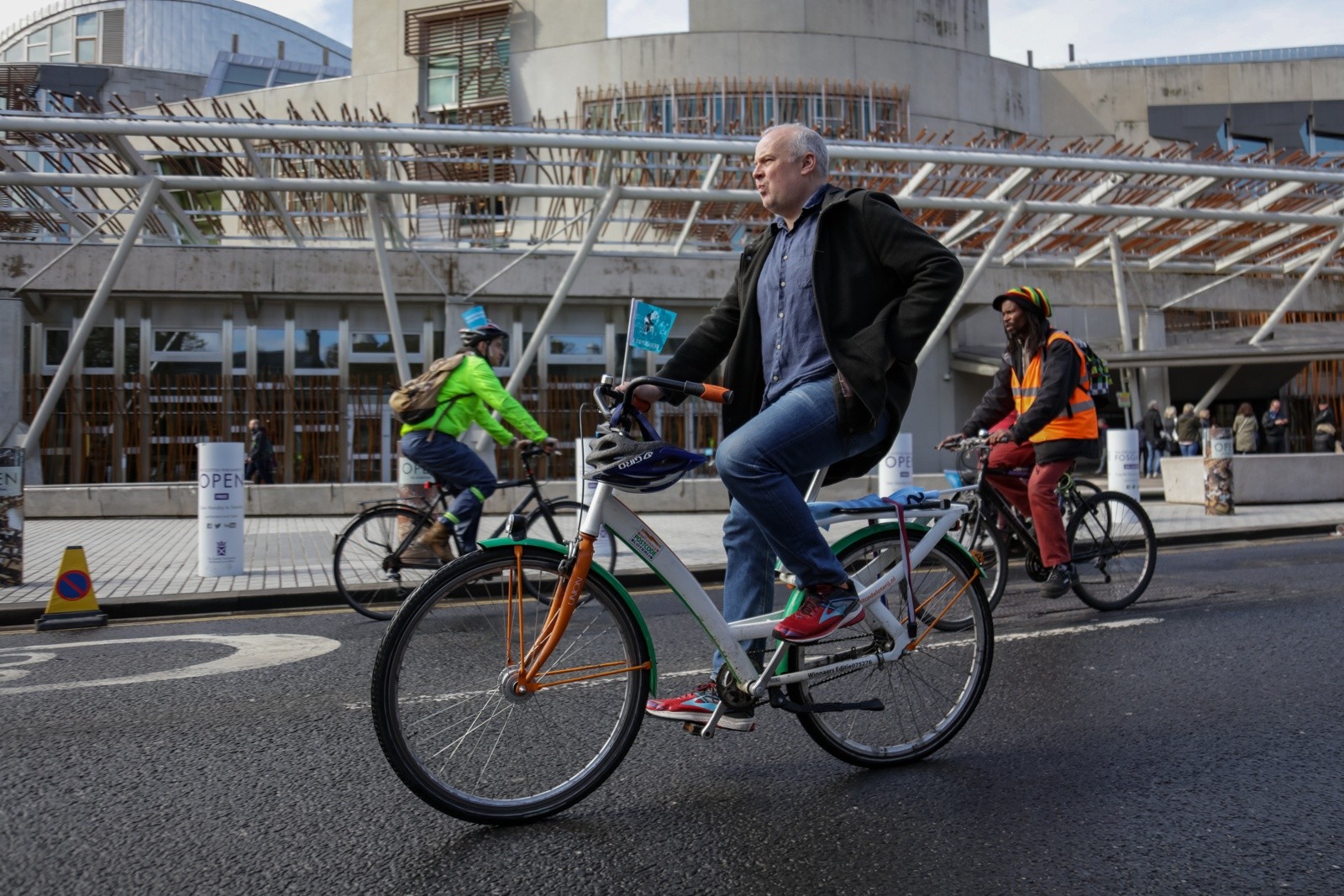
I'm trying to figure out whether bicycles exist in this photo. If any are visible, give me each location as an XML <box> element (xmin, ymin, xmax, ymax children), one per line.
<box><xmin>897</xmin><ymin>428</ymin><xmax>1160</xmax><ymax>631</ymax></box>
<box><xmin>330</xmin><ymin>441</ymin><xmax>620</xmax><ymax>622</ymax></box>
<box><xmin>367</xmin><ymin>378</ymin><xmax>1002</xmax><ymax>831</ymax></box>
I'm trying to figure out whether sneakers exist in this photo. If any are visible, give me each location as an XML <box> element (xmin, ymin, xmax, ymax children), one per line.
<box><xmin>1041</xmin><ymin>565</ymin><xmax>1071</xmax><ymax>598</ymax></box>
<box><xmin>772</xmin><ymin>580</ymin><xmax>864</xmax><ymax>643</ymax></box>
<box><xmin>646</xmin><ymin>683</ymin><xmax>757</xmax><ymax>732</ymax></box>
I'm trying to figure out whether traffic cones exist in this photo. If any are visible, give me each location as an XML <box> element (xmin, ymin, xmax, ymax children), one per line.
<box><xmin>32</xmin><ymin>547</ymin><xmax>111</xmax><ymax>635</ymax></box>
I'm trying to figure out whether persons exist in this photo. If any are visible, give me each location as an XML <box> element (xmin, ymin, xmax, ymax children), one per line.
<box><xmin>938</xmin><ymin>286</ymin><xmax>1097</xmax><ymax>598</ymax></box>
<box><xmin>1095</xmin><ymin>419</ymin><xmax>1110</xmax><ymax>476</ymax></box>
<box><xmin>1136</xmin><ymin>398</ymin><xmax>1215</xmax><ymax>479</ymax></box>
<box><xmin>246</xmin><ymin>419</ymin><xmax>274</xmax><ymax>484</ymax></box>
<box><xmin>610</xmin><ymin>122</ymin><xmax>963</xmax><ymax>729</ymax></box>
<box><xmin>1314</xmin><ymin>399</ymin><xmax>1336</xmax><ymax>453</ymax></box>
<box><xmin>400</xmin><ymin>325</ymin><xmax>559</xmax><ymax>581</ymax></box>
<box><xmin>1262</xmin><ymin>400</ymin><xmax>1288</xmax><ymax>453</ymax></box>
<box><xmin>1233</xmin><ymin>403</ymin><xmax>1258</xmax><ymax>454</ymax></box>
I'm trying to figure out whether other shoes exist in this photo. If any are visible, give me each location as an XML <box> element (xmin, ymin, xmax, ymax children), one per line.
<box><xmin>416</xmin><ymin>520</ymin><xmax>457</xmax><ymax>567</ymax></box>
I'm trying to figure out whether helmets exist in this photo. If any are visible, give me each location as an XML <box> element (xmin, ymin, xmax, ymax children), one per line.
<box><xmin>583</xmin><ymin>394</ymin><xmax>710</xmax><ymax>495</ymax></box>
<box><xmin>459</xmin><ymin>321</ymin><xmax>509</xmax><ymax>346</ymax></box>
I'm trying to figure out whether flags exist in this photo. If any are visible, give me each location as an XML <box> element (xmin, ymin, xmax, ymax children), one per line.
<box><xmin>629</xmin><ymin>301</ymin><xmax>677</xmax><ymax>355</ymax></box>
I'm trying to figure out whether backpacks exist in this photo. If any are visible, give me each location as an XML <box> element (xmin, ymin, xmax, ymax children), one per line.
<box><xmin>1045</xmin><ymin>329</ymin><xmax>1114</xmax><ymax>402</ymax></box>
<box><xmin>389</xmin><ymin>352</ymin><xmax>475</xmax><ymax>425</ymax></box>
